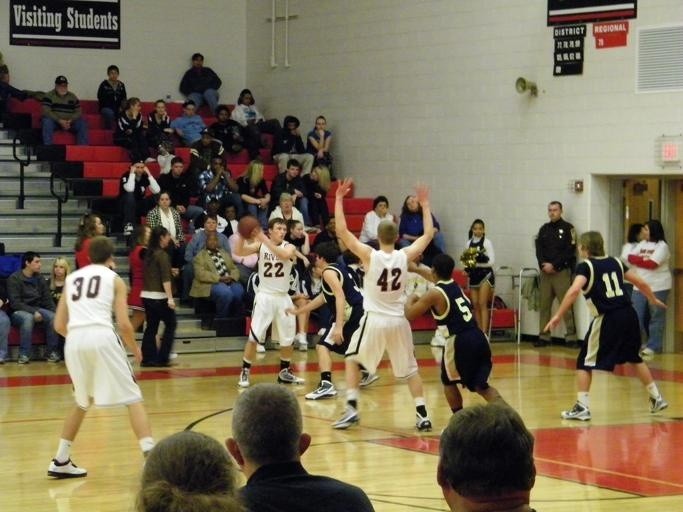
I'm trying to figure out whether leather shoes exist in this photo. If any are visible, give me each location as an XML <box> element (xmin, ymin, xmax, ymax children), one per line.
<box><xmin>534</xmin><ymin>339</ymin><xmax>552</xmax><ymax>347</ymax></box>
<box><xmin>566</xmin><ymin>341</ymin><xmax>577</xmax><ymax>349</ymax></box>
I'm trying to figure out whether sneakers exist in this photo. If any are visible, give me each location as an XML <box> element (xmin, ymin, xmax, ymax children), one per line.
<box><xmin>331</xmin><ymin>406</ymin><xmax>360</xmax><ymax>429</ymax></box>
<box><xmin>642</xmin><ymin>348</ymin><xmax>654</xmax><ymax>355</ymax></box>
<box><xmin>304</xmin><ymin>380</ymin><xmax>337</xmax><ymax>400</ymax></box>
<box><xmin>359</xmin><ymin>373</ymin><xmax>380</xmax><ymax>387</ymax></box>
<box><xmin>47</xmin><ymin>352</ymin><xmax>60</xmax><ymax>362</ymax></box>
<box><xmin>168</xmin><ymin>353</ymin><xmax>178</xmax><ymax>359</ymax></box>
<box><xmin>415</xmin><ymin>410</ymin><xmax>432</xmax><ymax>432</ymax></box>
<box><xmin>256</xmin><ymin>343</ymin><xmax>265</xmax><ymax>353</ymax></box>
<box><xmin>277</xmin><ymin>368</ymin><xmax>305</xmax><ymax>385</ymax></box>
<box><xmin>237</xmin><ymin>367</ymin><xmax>250</xmax><ymax>388</ymax></box>
<box><xmin>560</xmin><ymin>403</ymin><xmax>591</xmax><ymax>421</ymax></box>
<box><xmin>430</xmin><ymin>335</ymin><xmax>446</xmax><ymax>347</ymax></box>
<box><xmin>17</xmin><ymin>354</ymin><xmax>29</xmax><ymax>364</ymax></box>
<box><xmin>648</xmin><ymin>395</ymin><xmax>668</xmax><ymax>414</ymax></box>
<box><xmin>47</xmin><ymin>457</ymin><xmax>87</xmax><ymax>477</ymax></box>
<box><xmin>295</xmin><ymin>333</ymin><xmax>308</xmax><ymax>351</ymax></box>
<box><xmin>318</xmin><ymin>327</ymin><xmax>326</xmax><ymax>336</ymax></box>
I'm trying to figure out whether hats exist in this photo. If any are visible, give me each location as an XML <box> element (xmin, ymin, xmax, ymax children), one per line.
<box><xmin>181</xmin><ymin>99</ymin><xmax>195</xmax><ymax>108</ymax></box>
<box><xmin>55</xmin><ymin>76</ymin><xmax>68</xmax><ymax>84</ymax></box>
<box><xmin>129</xmin><ymin>158</ymin><xmax>144</xmax><ymax>169</ymax></box>
<box><xmin>199</xmin><ymin>127</ymin><xmax>215</xmax><ymax>136</ymax></box>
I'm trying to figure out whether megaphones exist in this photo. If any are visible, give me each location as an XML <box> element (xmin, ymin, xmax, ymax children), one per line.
<box><xmin>515</xmin><ymin>76</ymin><xmax>536</xmax><ymax>94</ymax></box>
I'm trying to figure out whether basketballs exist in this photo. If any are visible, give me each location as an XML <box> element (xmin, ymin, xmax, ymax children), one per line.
<box><xmin>237</xmin><ymin>216</ymin><xmax>260</xmax><ymax>239</ymax></box>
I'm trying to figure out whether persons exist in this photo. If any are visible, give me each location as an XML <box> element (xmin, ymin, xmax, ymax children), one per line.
<box><xmin>230</xmin><ymin>89</ymin><xmax>281</xmax><ymax>148</ymax></box>
<box><xmin>437</xmin><ymin>402</ymin><xmax>537</xmax><ymax>512</ymax></box>
<box><xmin>170</xmin><ymin>100</ymin><xmax>207</xmax><ymax>147</ymax></box>
<box><xmin>330</xmin><ymin>177</ymin><xmax>434</xmax><ymax>432</ymax></box>
<box><xmin>272</xmin><ymin>115</ymin><xmax>314</xmax><ymax>178</ymax></box>
<box><xmin>195</xmin><ymin>197</ymin><xmax>228</xmax><ymax>233</ymax></box>
<box><xmin>7</xmin><ymin>251</ymin><xmax>61</xmax><ymax>362</ymax></box>
<box><xmin>120</xmin><ymin>225</ymin><xmax>177</xmax><ymax>360</ymax></box>
<box><xmin>235</xmin><ymin>217</ymin><xmax>306</xmax><ymax>388</ymax></box>
<box><xmin>179</xmin><ymin>53</ymin><xmax>221</xmax><ymax>115</ymax></box>
<box><xmin>113</xmin><ymin>97</ymin><xmax>156</xmax><ymax>163</ymax></box>
<box><xmin>189</xmin><ymin>235</ymin><xmax>245</xmax><ymax>319</ymax></box>
<box><xmin>621</xmin><ymin>223</ymin><xmax>651</xmax><ymax>349</ymax></box>
<box><xmin>206</xmin><ymin>104</ymin><xmax>265</xmax><ymax>162</ymax></box>
<box><xmin>224</xmin><ymin>382</ymin><xmax>374</xmax><ymax>512</ymax></box>
<box><xmin>40</xmin><ymin>75</ymin><xmax>87</xmax><ymax>145</ymax></box>
<box><xmin>119</xmin><ymin>160</ymin><xmax>160</xmax><ymax>232</ymax></box>
<box><xmin>533</xmin><ymin>201</ymin><xmax>577</xmax><ymax>348</ymax></box>
<box><xmin>627</xmin><ymin>219</ymin><xmax>672</xmax><ymax>356</ymax></box>
<box><xmin>48</xmin><ymin>258</ymin><xmax>71</xmax><ymax>307</ymax></box>
<box><xmin>135</xmin><ymin>432</ymin><xmax>250</xmax><ymax>511</ymax></box>
<box><xmin>157</xmin><ymin>156</ymin><xmax>204</xmax><ymax>230</ymax></box>
<box><xmin>97</xmin><ymin>65</ymin><xmax>128</xmax><ymax>122</ymax></box>
<box><xmin>145</xmin><ymin>189</ymin><xmax>185</xmax><ymax>248</ymax></box>
<box><xmin>227</xmin><ymin>232</ymin><xmax>269</xmax><ymax>285</ymax></box>
<box><xmin>313</xmin><ymin>215</ymin><xmax>339</xmax><ymax>252</ymax></box>
<box><xmin>221</xmin><ymin>203</ymin><xmax>240</xmax><ymax>238</ymax></box>
<box><xmin>74</xmin><ymin>214</ymin><xmax>116</xmax><ymax>270</ymax></box>
<box><xmin>396</xmin><ymin>195</ymin><xmax>447</xmax><ymax>254</ymax></box>
<box><xmin>198</xmin><ymin>157</ymin><xmax>244</xmax><ymax>221</ymax></box>
<box><xmin>267</xmin><ymin>158</ymin><xmax>303</xmax><ymax>219</ymax></box>
<box><xmin>285</xmin><ymin>220</ymin><xmax>311</xmax><ymax>285</ymax></box>
<box><xmin>147</xmin><ymin>99</ymin><xmax>173</xmax><ymax>154</ymax></box>
<box><xmin>0</xmin><ymin>277</ymin><xmax>10</xmax><ymax>363</ymax></box>
<box><xmin>48</xmin><ymin>236</ymin><xmax>155</xmax><ymax>478</ymax></box>
<box><xmin>404</xmin><ymin>254</ymin><xmax>507</xmax><ymax>435</ymax></box>
<box><xmin>184</xmin><ymin>213</ymin><xmax>231</xmax><ymax>258</ymax></box>
<box><xmin>465</xmin><ymin>219</ymin><xmax>496</xmax><ymax>344</ymax></box>
<box><xmin>268</xmin><ymin>192</ymin><xmax>304</xmax><ymax>231</ymax></box>
<box><xmin>284</xmin><ymin>244</ymin><xmax>380</xmax><ymax>400</ymax></box>
<box><xmin>301</xmin><ymin>165</ymin><xmax>331</xmax><ymax>225</ymax></box>
<box><xmin>0</xmin><ymin>52</ymin><xmax>28</xmax><ymax>129</ymax></box>
<box><xmin>307</xmin><ymin>115</ymin><xmax>337</xmax><ymax>182</ymax></box>
<box><xmin>359</xmin><ymin>195</ymin><xmax>400</xmax><ymax>248</ymax></box>
<box><xmin>544</xmin><ymin>232</ymin><xmax>668</xmax><ymax>421</ymax></box>
<box><xmin>189</xmin><ymin>127</ymin><xmax>224</xmax><ymax>164</ymax></box>
<box><xmin>139</xmin><ymin>226</ymin><xmax>179</xmax><ymax>367</ymax></box>
<box><xmin>236</xmin><ymin>159</ymin><xmax>271</xmax><ymax>230</ymax></box>
